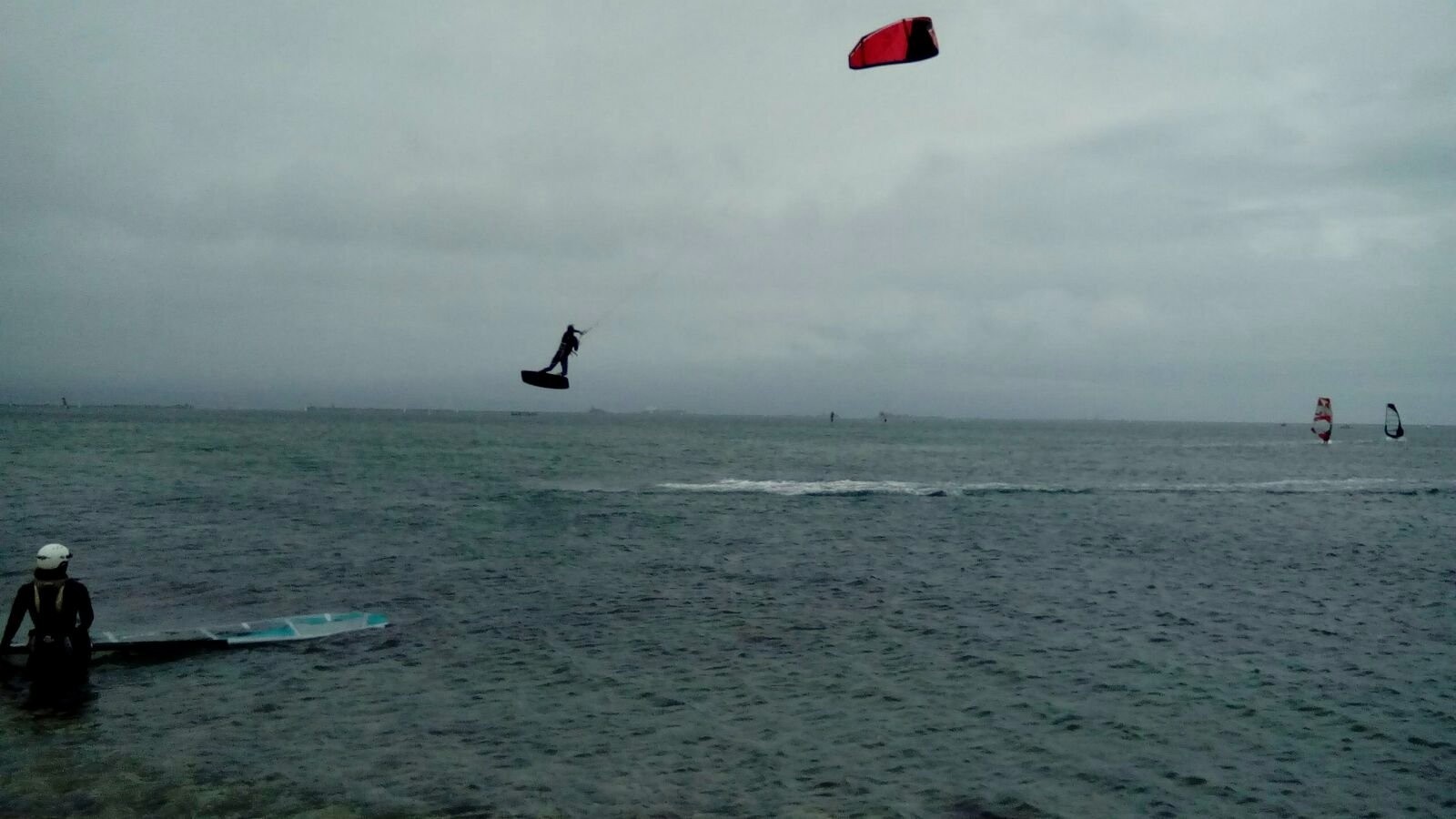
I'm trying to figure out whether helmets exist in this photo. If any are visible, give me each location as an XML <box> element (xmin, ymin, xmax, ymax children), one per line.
<box><xmin>36</xmin><ymin>543</ymin><xmax>72</xmax><ymax>569</ymax></box>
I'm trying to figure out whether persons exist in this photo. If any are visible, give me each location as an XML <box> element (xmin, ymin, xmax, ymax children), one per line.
<box><xmin>0</xmin><ymin>544</ymin><xmax>95</xmax><ymax>676</ymax></box>
<box><xmin>539</xmin><ymin>325</ymin><xmax>583</xmax><ymax>377</ymax></box>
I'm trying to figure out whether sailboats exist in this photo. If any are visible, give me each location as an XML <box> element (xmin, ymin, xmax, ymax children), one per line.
<box><xmin>1385</xmin><ymin>403</ymin><xmax>1404</xmax><ymax>440</ymax></box>
<box><xmin>1312</xmin><ymin>397</ymin><xmax>1332</xmax><ymax>443</ymax></box>
